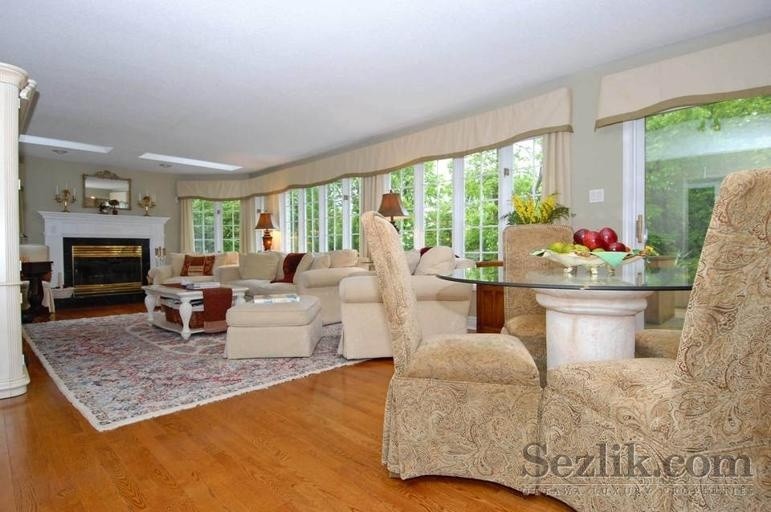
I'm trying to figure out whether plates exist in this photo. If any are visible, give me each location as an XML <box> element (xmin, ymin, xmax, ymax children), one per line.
<box><xmin>532</xmin><ymin>249</ymin><xmax>642</xmax><ymax>275</ymax></box>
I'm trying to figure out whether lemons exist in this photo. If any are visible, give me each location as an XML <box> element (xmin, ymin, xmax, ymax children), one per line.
<box><xmin>548</xmin><ymin>241</ymin><xmax>591</xmax><ymax>257</ymax></box>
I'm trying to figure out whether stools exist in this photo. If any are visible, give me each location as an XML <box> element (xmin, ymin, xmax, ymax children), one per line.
<box><xmin>223</xmin><ymin>292</ymin><xmax>324</xmax><ymax>359</ymax></box>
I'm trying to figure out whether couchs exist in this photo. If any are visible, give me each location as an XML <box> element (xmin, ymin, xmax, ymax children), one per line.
<box><xmin>338</xmin><ymin>245</ymin><xmax>475</xmax><ymax>362</ymax></box>
<box><xmin>216</xmin><ymin>248</ymin><xmax>366</xmax><ymax>318</ymax></box>
<box><xmin>146</xmin><ymin>251</ymin><xmax>238</xmax><ymax>289</ymax></box>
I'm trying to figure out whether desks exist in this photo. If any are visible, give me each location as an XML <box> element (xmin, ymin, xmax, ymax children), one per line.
<box><xmin>435</xmin><ymin>246</ymin><xmax>699</xmax><ymax>362</ymax></box>
<box><xmin>20</xmin><ymin>261</ymin><xmax>52</xmax><ymax>320</ymax></box>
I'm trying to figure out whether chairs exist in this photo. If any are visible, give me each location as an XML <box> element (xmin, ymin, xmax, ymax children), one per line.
<box><xmin>536</xmin><ymin>167</ymin><xmax>771</xmax><ymax>512</ymax></box>
<box><xmin>499</xmin><ymin>222</ymin><xmax>578</xmax><ymax>377</ymax></box>
<box><xmin>361</xmin><ymin>209</ymin><xmax>544</xmax><ymax>497</ymax></box>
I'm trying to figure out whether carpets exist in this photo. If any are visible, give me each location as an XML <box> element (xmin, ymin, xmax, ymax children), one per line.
<box><xmin>21</xmin><ymin>308</ymin><xmax>374</xmax><ymax>432</ymax></box>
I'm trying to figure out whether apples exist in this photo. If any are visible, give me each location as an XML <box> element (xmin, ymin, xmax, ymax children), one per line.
<box><xmin>573</xmin><ymin>227</ymin><xmax>626</xmax><ymax>252</ymax></box>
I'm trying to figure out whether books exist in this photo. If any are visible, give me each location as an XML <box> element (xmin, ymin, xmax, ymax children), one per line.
<box><xmin>254</xmin><ymin>294</ymin><xmax>299</xmax><ymax>305</ymax></box>
<box><xmin>187</xmin><ymin>282</ymin><xmax>219</xmax><ymax>290</ymax></box>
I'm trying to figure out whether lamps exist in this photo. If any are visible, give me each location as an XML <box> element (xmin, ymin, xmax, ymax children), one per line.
<box><xmin>55</xmin><ymin>182</ymin><xmax>77</xmax><ymax>212</ymax></box>
<box><xmin>137</xmin><ymin>187</ymin><xmax>159</xmax><ymax>217</ymax></box>
<box><xmin>374</xmin><ymin>193</ymin><xmax>410</xmax><ymax>236</ymax></box>
<box><xmin>255</xmin><ymin>212</ymin><xmax>280</xmax><ymax>252</ymax></box>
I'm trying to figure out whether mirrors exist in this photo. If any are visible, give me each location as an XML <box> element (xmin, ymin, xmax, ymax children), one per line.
<box><xmin>82</xmin><ymin>172</ymin><xmax>133</xmax><ymax>212</ymax></box>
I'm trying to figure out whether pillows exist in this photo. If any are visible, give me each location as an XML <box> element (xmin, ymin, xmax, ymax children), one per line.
<box><xmin>237</xmin><ymin>253</ymin><xmax>331</xmax><ymax>283</ymax></box>
<box><xmin>181</xmin><ymin>253</ymin><xmax>216</xmax><ymax>277</ymax></box>
